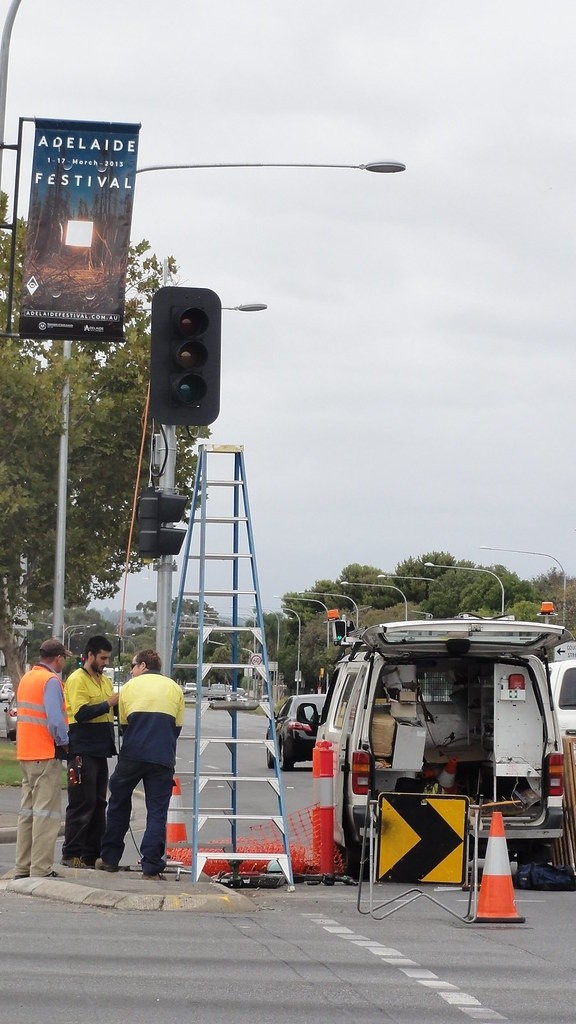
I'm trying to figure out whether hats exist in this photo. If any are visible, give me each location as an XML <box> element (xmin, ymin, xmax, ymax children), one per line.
<box><xmin>39</xmin><ymin>640</ymin><xmax>73</xmax><ymax>658</ymax></box>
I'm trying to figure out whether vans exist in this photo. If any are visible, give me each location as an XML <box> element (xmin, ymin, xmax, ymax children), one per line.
<box><xmin>545</xmin><ymin>660</ymin><xmax>576</xmax><ymax>743</ymax></box>
<box><xmin>311</xmin><ymin>618</ymin><xmax>565</xmax><ymax>881</ymax></box>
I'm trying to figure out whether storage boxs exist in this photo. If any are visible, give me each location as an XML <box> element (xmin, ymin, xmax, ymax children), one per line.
<box><xmin>371</xmin><ymin>665</ymin><xmax>427</xmax><ymax>791</ymax></box>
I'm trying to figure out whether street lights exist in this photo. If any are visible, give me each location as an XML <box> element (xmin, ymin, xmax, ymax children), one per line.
<box><xmin>424</xmin><ymin>562</ymin><xmax>505</xmax><ymax>616</ymax></box>
<box><xmin>46</xmin><ymin>622</ymin><xmax>96</xmax><ymax>651</ymax></box>
<box><xmin>375</xmin><ymin>573</ymin><xmax>445</xmax><ymax>595</ymax></box>
<box><xmin>479</xmin><ymin>546</ymin><xmax>566</xmax><ymax>626</ymax></box>
<box><xmin>52</xmin><ymin>161</ymin><xmax>407</xmax><ymax>644</ymax></box>
<box><xmin>158</xmin><ymin>301</ymin><xmax>269</xmax><ymax>680</ymax></box>
<box><xmin>296</xmin><ymin>591</ymin><xmax>358</xmax><ymax>630</ymax></box>
<box><xmin>229</xmin><ymin>604</ymin><xmax>301</xmax><ymax>702</ymax></box>
<box><xmin>340</xmin><ymin>581</ymin><xmax>408</xmax><ymax>621</ymax></box>
<box><xmin>273</xmin><ymin>595</ymin><xmax>329</xmax><ymax>649</ymax></box>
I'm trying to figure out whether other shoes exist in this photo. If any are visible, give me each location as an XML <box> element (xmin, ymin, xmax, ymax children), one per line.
<box><xmin>142</xmin><ymin>874</ymin><xmax>167</xmax><ymax>881</ymax></box>
<box><xmin>45</xmin><ymin>871</ymin><xmax>65</xmax><ymax>878</ymax></box>
<box><xmin>95</xmin><ymin>858</ymin><xmax>118</xmax><ymax>872</ymax></box>
<box><xmin>60</xmin><ymin>856</ymin><xmax>86</xmax><ymax>867</ymax></box>
<box><xmin>14</xmin><ymin>875</ymin><xmax>29</xmax><ymax>880</ymax></box>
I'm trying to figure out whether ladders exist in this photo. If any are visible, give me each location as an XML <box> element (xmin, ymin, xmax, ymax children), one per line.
<box><xmin>169</xmin><ymin>444</ymin><xmax>295</xmax><ymax>894</ymax></box>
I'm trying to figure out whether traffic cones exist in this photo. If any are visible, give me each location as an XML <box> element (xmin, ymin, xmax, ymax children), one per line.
<box><xmin>468</xmin><ymin>812</ymin><xmax>527</xmax><ymax>922</ymax></box>
<box><xmin>163</xmin><ymin>776</ymin><xmax>192</xmax><ymax>861</ymax></box>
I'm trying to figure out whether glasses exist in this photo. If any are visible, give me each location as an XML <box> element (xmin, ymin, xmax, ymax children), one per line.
<box><xmin>131</xmin><ymin>663</ymin><xmax>138</xmax><ymax>669</ymax></box>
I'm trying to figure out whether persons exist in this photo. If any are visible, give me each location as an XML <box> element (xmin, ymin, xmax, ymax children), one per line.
<box><xmin>60</xmin><ymin>636</ymin><xmax>119</xmax><ymax>868</ymax></box>
<box><xmin>15</xmin><ymin>639</ymin><xmax>70</xmax><ymax>880</ymax></box>
<box><xmin>96</xmin><ymin>650</ymin><xmax>186</xmax><ymax>880</ymax></box>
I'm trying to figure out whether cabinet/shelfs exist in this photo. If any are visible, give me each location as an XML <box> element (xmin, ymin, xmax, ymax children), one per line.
<box><xmin>468</xmin><ymin>663</ymin><xmax>494</xmax><ymax>752</ymax></box>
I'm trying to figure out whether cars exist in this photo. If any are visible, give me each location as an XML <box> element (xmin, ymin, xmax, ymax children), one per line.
<box><xmin>266</xmin><ymin>694</ymin><xmax>327</xmax><ymax>772</ymax></box>
<box><xmin>0</xmin><ymin>675</ymin><xmax>16</xmax><ymax>704</ymax></box>
<box><xmin>183</xmin><ymin>682</ymin><xmax>249</xmax><ymax>704</ymax></box>
<box><xmin>3</xmin><ymin>695</ymin><xmax>18</xmax><ymax>742</ymax></box>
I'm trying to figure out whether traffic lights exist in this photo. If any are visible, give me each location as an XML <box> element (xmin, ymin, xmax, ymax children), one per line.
<box><xmin>333</xmin><ymin>620</ymin><xmax>347</xmax><ymax>645</ymax></box>
<box><xmin>156</xmin><ymin>491</ymin><xmax>189</xmax><ymax>556</ymax></box>
<box><xmin>151</xmin><ymin>286</ymin><xmax>220</xmax><ymax>426</ymax></box>
<box><xmin>77</xmin><ymin>655</ymin><xmax>85</xmax><ymax>666</ymax></box>
